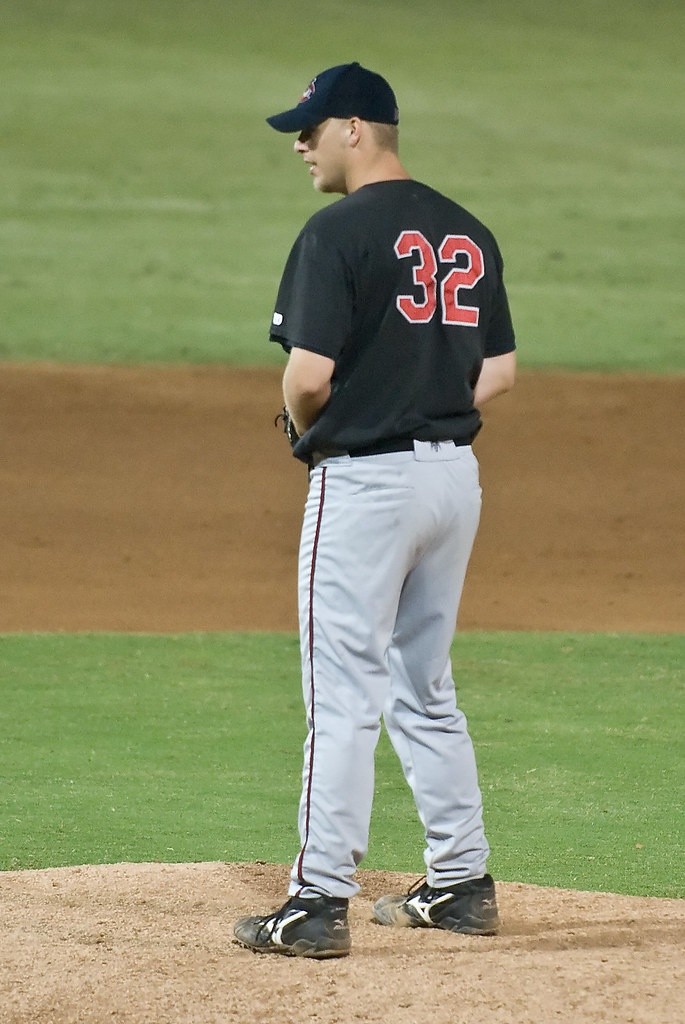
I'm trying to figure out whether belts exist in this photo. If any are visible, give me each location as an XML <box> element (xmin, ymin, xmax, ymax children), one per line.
<box><xmin>308</xmin><ymin>437</ymin><xmax>472</xmax><ymax>470</ymax></box>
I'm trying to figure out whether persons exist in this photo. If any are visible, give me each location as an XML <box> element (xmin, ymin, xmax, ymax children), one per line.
<box><xmin>232</xmin><ymin>64</ymin><xmax>517</xmax><ymax>956</ymax></box>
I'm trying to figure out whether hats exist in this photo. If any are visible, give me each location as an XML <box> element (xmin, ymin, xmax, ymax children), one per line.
<box><xmin>265</xmin><ymin>61</ymin><xmax>399</xmax><ymax>133</ymax></box>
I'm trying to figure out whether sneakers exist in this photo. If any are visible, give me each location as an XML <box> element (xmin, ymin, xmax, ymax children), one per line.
<box><xmin>233</xmin><ymin>897</ymin><xmax>352</xmax><ymax>957</ymax></box>
<box><xmin>371</xmin><ymin>874</ymin><xmax>501</xmax><ymax>935</ymax></box>
